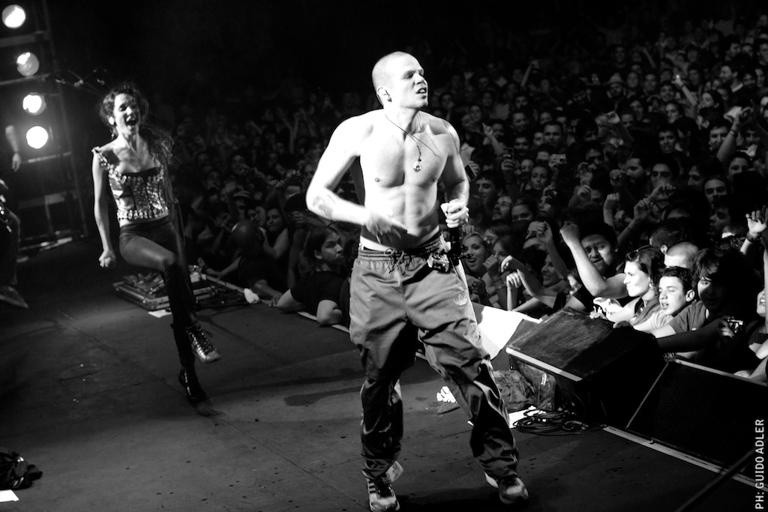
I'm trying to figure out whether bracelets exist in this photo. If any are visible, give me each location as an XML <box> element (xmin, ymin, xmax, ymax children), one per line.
<box><xmin>13</xmin><ymin>150</ymin><xmax>20</xmax><ymax>154</ymax></box>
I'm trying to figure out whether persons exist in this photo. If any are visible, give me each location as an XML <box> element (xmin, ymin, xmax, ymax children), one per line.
<box><xmin>300</xmin><ymin>43</ymin><xmax>531</xmax><ymax>509</ymax></box>
<box><xmin>0</xmin><ymin>96</ymin><xmax>30</xmax><ymax>311</ymax></box>
<box><xmin>148</xmin><ymin>2</ymin><xmax>767</xmax><ymax>386</ymax></box>
<box><xmin>87</xmin><ymin>81</ymin><xmax>224</xmax><ymax>418</ymax></box>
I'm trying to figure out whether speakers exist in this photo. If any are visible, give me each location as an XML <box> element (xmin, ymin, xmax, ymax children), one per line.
<box><xmin>506</xmin><ymin>303</ymin><xmax>668</xmax><ymax>427</ymax></box>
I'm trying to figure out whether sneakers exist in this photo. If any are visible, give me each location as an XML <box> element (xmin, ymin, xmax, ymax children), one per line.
<box><xmin>187</xmin><ymin>323</ymin><xmax>222</xmax><ymax>365</ymax></box>
<box><xmin>1</xmin><ymin>285</ymin><xmax>28</xmax><ymax>308</ymax></box>
<box><xmin>178</xmin><ymin>368</ymin><xmax>209</xmax><ymax>405</ymax></box>
<box><xmin>485</xmin><ymin>470</ymin><xmax>531</xmax><ymax>505</ymax></box>
<box><xmin>366</xmin><ymin>474</ymin><xmax>399</xmax><ymax>512</ymax></box>
